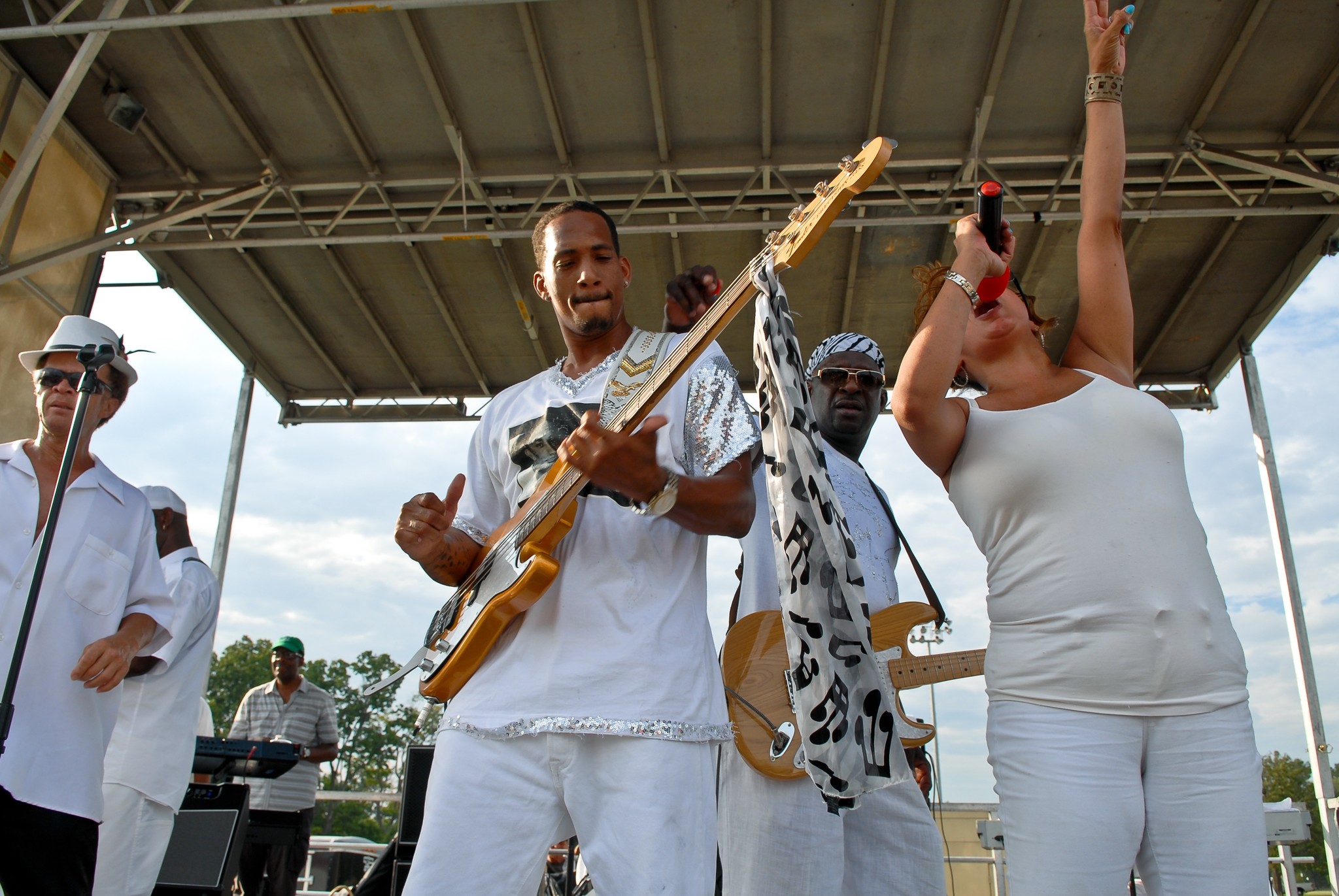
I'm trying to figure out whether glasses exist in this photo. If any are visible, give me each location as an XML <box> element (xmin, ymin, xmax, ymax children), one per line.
<box><xmin>274</xmin><ymin>651</ymin><xmax>302</xmax><ymax>660</ymax></box>
<box><xmin>34</xmin><ymin>368</ymin><xmax>114</xmax><ymax>395</ymax></box>
<box><xmin>812</xmin><ymin>367</ymin><xmax>886</xmax><ymax>391</ymax></box>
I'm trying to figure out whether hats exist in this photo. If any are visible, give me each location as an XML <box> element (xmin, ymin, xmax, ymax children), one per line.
<box><xmin>138</xmin><ymin>486</ymin><xmax>186</xmax><ymax>518</ymax></box>
<box><xmin>18</xmin><ymin>315</ymin><xmax>138</xmax><ymax>388</ymax></box>
<box><xmin>804</xmin><ymin>332</ymin><xmax>885</xmax><ymax>379</ymax></box>
<box><xmin>271</xmin><ymin>637</ymin><xmax>304</xmax><ymax>656</ymax></box>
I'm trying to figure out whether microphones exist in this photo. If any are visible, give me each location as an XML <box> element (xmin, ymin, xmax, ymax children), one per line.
<box><xmin>976</xmin><ymin>181</ymin><xmax>1011</xmax><ymax>302</ymax></box>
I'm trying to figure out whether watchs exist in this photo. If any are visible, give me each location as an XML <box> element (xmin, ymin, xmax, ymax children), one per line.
<box><xmin>303</xmin><ymin>747</ymin><xmax>310</xmax><ymax>758</ymax></box>
<box><xmin>628</xmin><ymin>467</ymin><xmax>679</xmax><ymax>517</ymax></box>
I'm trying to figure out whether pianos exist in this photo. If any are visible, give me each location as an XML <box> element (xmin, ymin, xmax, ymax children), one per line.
<box><xmin>190</xmin><ymin>735</ymin><xmax>306</xmax><ymax>781</ymax></box>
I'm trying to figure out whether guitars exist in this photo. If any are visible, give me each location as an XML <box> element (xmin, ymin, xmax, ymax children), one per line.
<box><xmin>720</xmin><ymin>601</ymin><xmax>987</xmax><ymax>782</ymax></box>
<box><xmin>419</xmin><ymin>135</ymin><xmax>898</xmax><ymax>705</ymax></box>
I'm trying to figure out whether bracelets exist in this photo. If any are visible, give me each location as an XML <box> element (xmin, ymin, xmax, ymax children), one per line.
<box><xmin>1084</xmin><ymin>73</ymin><xmax>1124</xmax><ymax>105</ymax></box>
<box><xmin>943</xmin><ymin>270</ymin><xmax>980</xmax><ymax>305</ymax></box>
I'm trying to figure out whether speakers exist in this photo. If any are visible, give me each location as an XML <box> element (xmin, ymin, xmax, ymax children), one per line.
<box><xmin>390</xmin><ymin>746</ymin><xmax>436</xmax><ymax>896</ymax></box>
<box><xmin>150</xmin><ymin>783</ymin><xmax>251</xmax><ymax>896</ymax></box>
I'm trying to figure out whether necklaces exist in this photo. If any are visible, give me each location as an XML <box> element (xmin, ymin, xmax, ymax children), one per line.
<box><xmin>550</xmin><ymin>350</ymin><xmax>621</xmax><ymax>397</ymax></box>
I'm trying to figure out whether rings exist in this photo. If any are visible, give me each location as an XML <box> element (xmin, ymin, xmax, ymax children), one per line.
<box><xmin>409</xmin><ymin>520</ymin><xmax>417</xmax><ymax>528</ymax></box>
<box><xmin>1109</xmin><ymin>17</ymin><xmax>1112</xmax><ymax>24</ymax></box>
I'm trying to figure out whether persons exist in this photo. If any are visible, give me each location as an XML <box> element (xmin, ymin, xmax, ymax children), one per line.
<box><xmin>189</xmin><ymin>697</ymin><xmax>214</xmax><ymax>782</ymax></box>
<box><xmin>92</xmin><ymin>486</ymin><xmax>220</xmax><ymax>896</ymax></box>
<box><xmin>391</xmin><ymin>201</ymin><xmax>766</xmax><ymax>896</ymax></box>
<box><xmin>226</xmin><ymin>637</ymin><xmax>338</xmax><ymax>896</ymax></box>
<box><xmin>518</xmin><ymin>417</ymin><xmax>560</xmax><ymax>503</ymax></box>
<box><xmin>890</xmin><ymin>0</ymin><xmax>1268</xmax><ymax>896</ymax></box>
<box><xmin>662</xmin><ymin>265</ymin><xmax>947</xmax><ymax>896</ymax></box>
<box><xmin>0</xmin><ymin>315</ymin><xmax>177</xmax><ymax>896</ymax></box>
